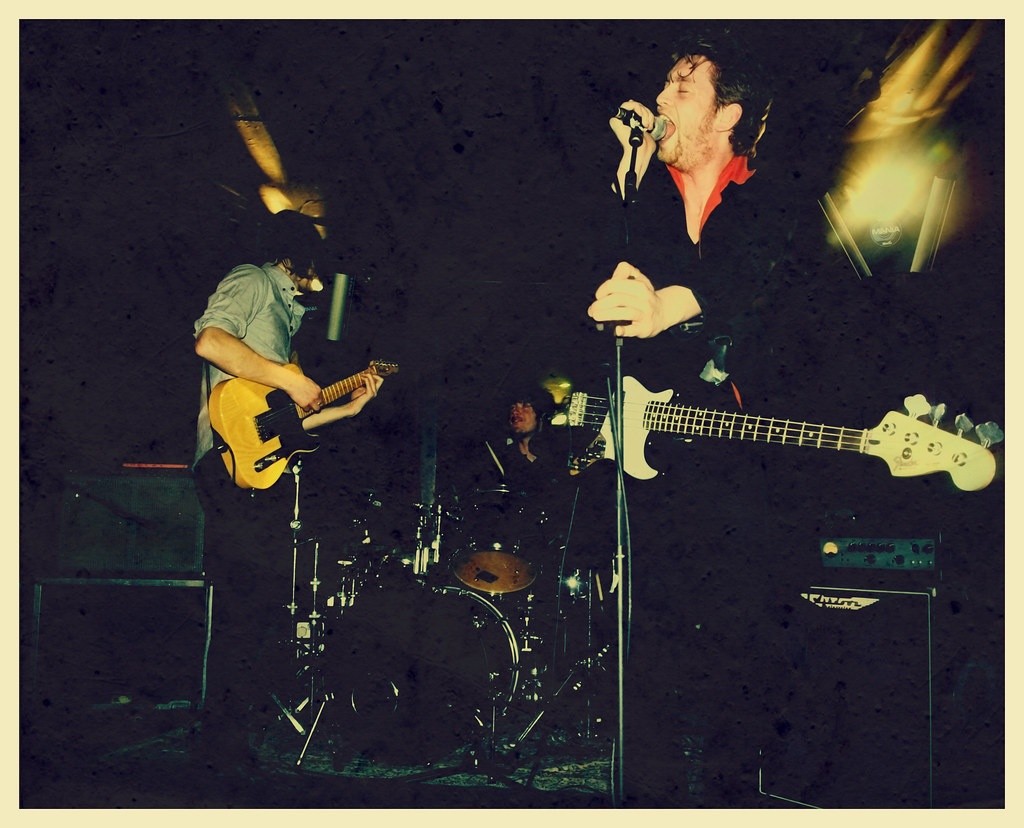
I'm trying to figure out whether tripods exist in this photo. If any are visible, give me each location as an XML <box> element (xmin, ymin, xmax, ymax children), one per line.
<box><xmin>498</xmin><ymin>563</ymin><xmax>613</xmax><ymax>757</ymax></box>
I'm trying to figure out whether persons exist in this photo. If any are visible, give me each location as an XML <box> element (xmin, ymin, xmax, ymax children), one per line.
<box><xmin>192</xmin><ymin>209</ymin><xmax>384</xmax><ymax>724</ymax></box>
<box><xmin>487</xmin><ymin>386</ymin><xmax>555</xmax><ymax>473</ymax></box>
<box><xmin>587</xmin><ymin>30</ymin><xmax>836</xmax><ymax>412</ymax></box>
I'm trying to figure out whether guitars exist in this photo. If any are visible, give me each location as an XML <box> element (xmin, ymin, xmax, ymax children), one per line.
<box><xmin>556</xmin><ymin>359</ymin><xmax>1004</xmax><ymax>505</ymax></box>
<box><xmin>208</xmin><ymin>358</ymin><xmax>401</xmax><ymax>490</ymax></box>
<box><xmin>663</xmin><ymin>407</ymin><xmax>673</xmax><ymax>410</ymax></box>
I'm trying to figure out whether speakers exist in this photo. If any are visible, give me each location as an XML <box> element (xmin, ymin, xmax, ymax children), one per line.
<box><xmin>760</xmin><ymin>583</ymin><xmax>981</xmax><ymax>809</ymax></box>
<box><xmin>32</xmin><ymin>576</ymin><xmax>214</xmax><ymax>711</ymax></box>
<box><xmin>57</xmin><ymin>469</ymin><xmax>206</xmax><ymax>578</ymax></box>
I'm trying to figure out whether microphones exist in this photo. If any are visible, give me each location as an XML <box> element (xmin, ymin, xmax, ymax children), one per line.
<box><xmin>606</xmin><ymin>104</ymin><xmax>667</xmax><ymax>142</ymax></box>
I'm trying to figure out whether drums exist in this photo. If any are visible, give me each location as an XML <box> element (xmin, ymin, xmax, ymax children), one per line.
<box><xmin>336</xmin><ymin>569</ymin><xmax>529</xmax><ymax>765</ymax></box>
<box><xmin>445</xmin><ymin>478</ymin><xmax>549</xmax><ymax>597</ymax></box>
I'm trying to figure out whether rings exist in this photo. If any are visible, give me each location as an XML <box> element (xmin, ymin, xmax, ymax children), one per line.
<box><xmin>318</xmin><ymin>403</ymin><xmax>323</xmax><ymax>405</ymax></box>
